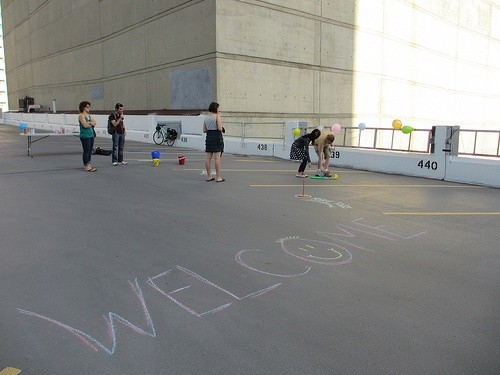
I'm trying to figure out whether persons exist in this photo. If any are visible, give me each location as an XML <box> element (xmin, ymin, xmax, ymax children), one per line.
<box><xmin>290</xmin><ymin>129</ymin><xmax>321</xmax><ymax>178</ymax></box>
<box><xmin>78</xmin><ymin>101</ymin><xmax>97</xmax><ymax>172</ymax></box>
<box><xmin>203</xmin><ymin>102</ymin><xmax>225</xmax><ymax>182</ymax></box>
<box><xmin>314</xmin><ymin>131</ymin><xmax>335</xmax><ymax>177</ymax></box>
<box><xmin>107</xmin><ymin>103</ymin><xmax>128</xmax><ymax>166</ymax></box>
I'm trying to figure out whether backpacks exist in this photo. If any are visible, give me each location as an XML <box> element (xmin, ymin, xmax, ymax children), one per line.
<box><xmin>108</xmin><ymin>112</ymin><xmax>116</xmax><ymax>135</ymax></box>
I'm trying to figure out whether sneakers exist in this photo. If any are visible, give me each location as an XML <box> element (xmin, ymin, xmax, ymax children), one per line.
<box><xmin>324</xmin><ymin>170</ymin><xmax>332</xmax><ymax>177</ymax></box>
<box><xmin>315</xmin><ymin>170</ymin><xmax>321</xmax><ymax>176</ymax></box>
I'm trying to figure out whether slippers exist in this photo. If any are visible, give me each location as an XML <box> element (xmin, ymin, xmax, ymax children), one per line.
<box><xmin>84</xmin><ymin>167</ymin><xmax>97</xmax><ymax>172</ymax></box>
<box><xmin>206</xmin><ymin>177</ymin><xmax>225</xmax><ymax>182</ymax></box>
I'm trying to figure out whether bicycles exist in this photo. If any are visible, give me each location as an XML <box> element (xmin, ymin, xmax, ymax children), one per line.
<box><xmin>153</xmin><ymin>123</ymin><xmax>176</xmax><ymax>146</ymax></box>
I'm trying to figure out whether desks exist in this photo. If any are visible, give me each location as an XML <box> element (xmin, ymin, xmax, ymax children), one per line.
<box><xmin>19</xmin><ymin>132</ymin><xmax>96</xmax><ymax>158</ymax></box>
<box><xmin>29</xmin><ymin>105</ymin><xmax>42</xmax><ymax>112</ymax></box>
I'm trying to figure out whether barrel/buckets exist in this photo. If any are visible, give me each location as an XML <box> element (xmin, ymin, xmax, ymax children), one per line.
<box><xmin>178</xmin><ymin>156</ymin><xmax>186</xmax><ymax>165</ymax></box>
<box><xmin>153</xmin><ymin>159</ymin><xmax>160</xmax><ymax>167</ymax></box>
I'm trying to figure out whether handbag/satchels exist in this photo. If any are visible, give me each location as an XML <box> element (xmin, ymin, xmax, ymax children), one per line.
<box><xmin>89</xmin><ymin>114</ymin><xmax>97</xmax><ymax>138</ymax></box>
<box><xmin>92</xmin><ymin>147</ymin><xmax>112</xmax><ymax>156</ymax></box>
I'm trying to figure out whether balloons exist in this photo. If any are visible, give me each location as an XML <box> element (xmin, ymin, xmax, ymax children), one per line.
<box><xmin>19</xmin><ymin>123</ymin><xmax>26</xmax><ymax>130</ymax></box>
<box><xmin>392</xmin><ymin>119</ymin><xmax>402</xmax><ymax>130</ymax></box>
<box><xmin>316</xmin><ymin>126</ymin><xmax>324</xmax><ymax>133</ymax></box>
<box><xmin>402</xmin><ymin>126</ymin><xmax>415</xmax><ymax>134</ymax></box>
<box><xmin>293</xmin><ymin>128</ymin><xmax>301</xmax><ymax>136</ymax></box>
<box><xmin>332</xmin><ymin>124</ymin><xmax>341</xmax><ymax>134</ymax></box>
<box><xmin>358</xmin><ymin>123</ymin><xmax>366</xmax><ymax>130</ymax></box>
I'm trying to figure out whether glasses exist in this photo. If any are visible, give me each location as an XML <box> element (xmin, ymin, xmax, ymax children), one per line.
<box><xmin>85</xmin><ymin>106</ymin><xmax>90</xmax><ymax>109</ymax></box>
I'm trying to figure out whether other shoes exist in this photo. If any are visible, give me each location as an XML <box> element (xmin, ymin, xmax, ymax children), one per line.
<box><xmin>113</xmin><ymin>161</ymin><xmax>128</xmax><ymax>166</ymax></box>
<box><xmin>296</xmin><ymin>173</ymin><xmax>308</xmax><ymax>178</ymax></box>
